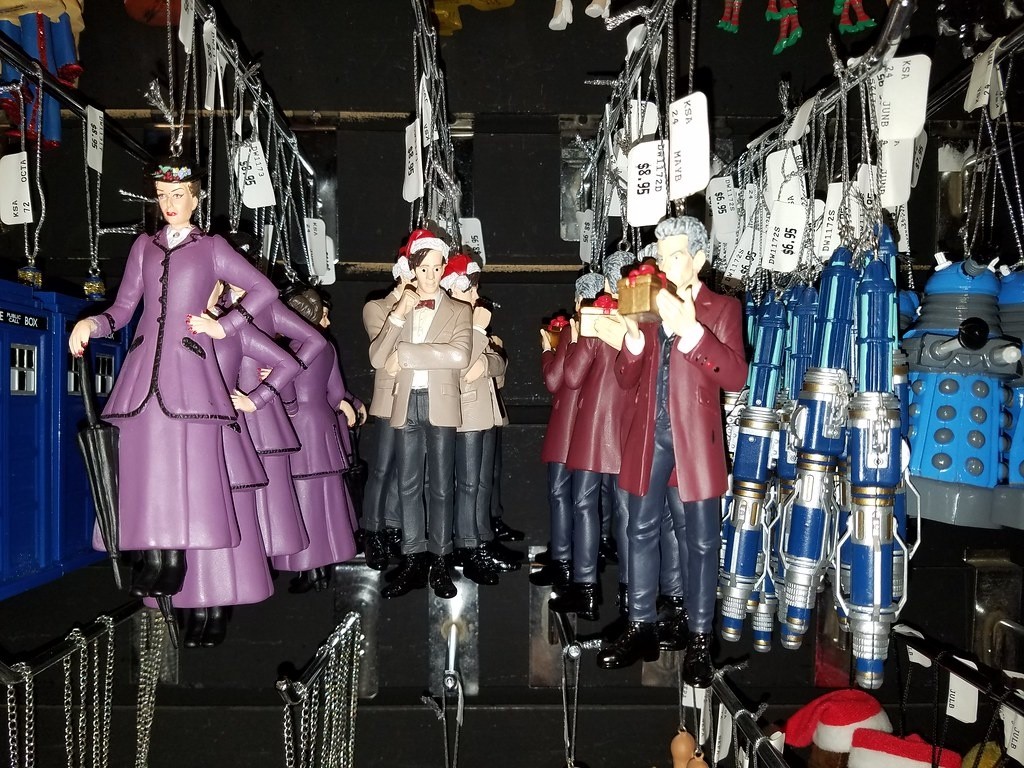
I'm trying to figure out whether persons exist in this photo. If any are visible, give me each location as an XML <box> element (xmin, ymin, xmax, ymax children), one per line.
<box><xmin>67</xmin><ymin>155</ymin><xmax>528</xmax><ymax>645</ymax></box>
<box><xmin>529</xmin><ymin>215</ymin><xmax>747</xmax><ymax>687</ymax></box>
